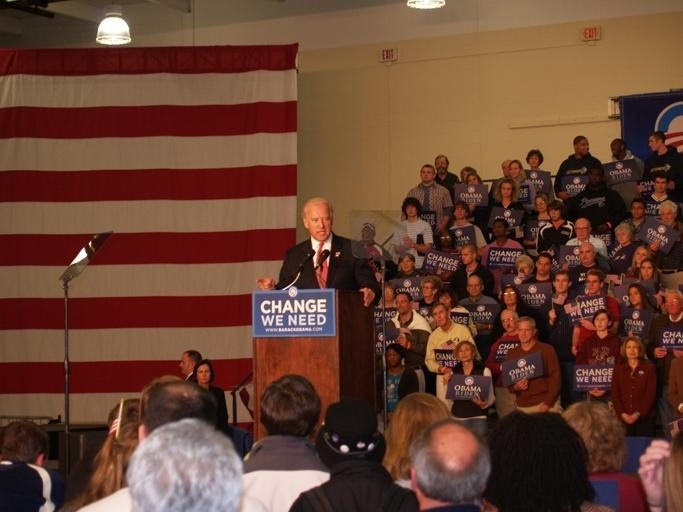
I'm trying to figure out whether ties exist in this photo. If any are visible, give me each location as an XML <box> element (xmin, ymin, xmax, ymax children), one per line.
<box><xmin>315</xmin><ymin>242</ymin><xmax>328</xmax><ymax>289</ymax></box>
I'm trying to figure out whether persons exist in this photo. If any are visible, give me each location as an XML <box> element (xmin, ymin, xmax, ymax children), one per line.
<box><xmin>534</xmin><ymin>200</ymin><xmax>575</xmax><ymax>251</ymax></box>
<box><xmin>455</xmin><ymin>164</ymin><xmax>480</xmax><ymax>188</ymax></box>
<box><xmin>499</xmin><ymin>284</ymin><xmax>525</xmax><ymax>310</ymax></box>
<box><xmin>256</xmin><ymin>196</ymin><xmax>382</xmax><ymax>306</ymax></box>
<box><xmin>645</xmin><ymin>131</ymin><xmax>683</xmax><ymax>201</ymax></box>
<box><xmin>557</xmin><ymin>135</ymin><xmax>605</xmax><ymax>200</ymax></box>
<box><xmin>571</xmin><ymin>267</ymin><xmax>621</xmax><ymax>353</ymax></box>
<box><xmin>667</xmin><ymin>355</ymin><xmax>683</xmax><ymax>439</ymax></box>
<box><xmin>59</xmin><ymin>375</ymin><xmax>243</xmax><ymax>512</ymax></box>
<box><xmin>394</xmin><ymin>288</ymin><xmax>430</xmax><ymax>357</ymax></box>
<box><xmin>564</xmin><ymin>217</ymin><xmax>608</xmax><ymax>265</ymax></box>
<box><xmin>377</xmin><ymin>344</ymin><xmax>418</xmax><ymax>428</ymax></box>
<box><xmin>457</xmin><ymin>172</ymin><xmax>485</xmax><ymax>205</ymax></box>
<box><xmin>434</xmin><ymin>155</ymin><xmax>460</xmax><ymax>202</ymax></box>
<box><xmin>524</xmin><ymin>192</ymin><xmax>552</xmax><ymax>225</ymax></box>
<box><xmin>610</xmin><ymin>337</ymin><xmax>658</xmax><ymax>435</ymax></box>
<box><xmin>494</xmin><ymin>409</ymin><xmax>601</xmax><ymax>510</ymax></box>
<box><xmin>401</xmin><ymin>164</ymin><xmax>454</xmax><ymax>234</ymax></box>
<box><xmin>608</xmin><ymin>222</ymin><xmax>644</xmax><ymax>272</ymax></box>
<box><xmin>645</xmin><ymin>171</ymin><xmax>680</xmax><ymax>220</ymax></box>
<box><xmin>487</xmin><ymin>181</ymin><xmax>530</xmax><ymax>228</ymax></box>
<box><xmin>180</xmin><ymin>350</ymin><xmax>229</xmax><ymax>423</ymax></box>
<box><xmin>381</xmin><ymin>390</ymin><xmax>450</xmax><ymax>489</ymax></box>
<box><xmin>495</xmin><ymin>162</ymin><xmax>537</xmax><ymax>212</ymax></box>
<box><xmin>479</xmin><ymin>217</ymin><xmax>528</xmax><ymax>295</ymax></box>
<box><xmin>505</xmin><ymin>318</ymin><xmax>562</xmax><ymax>413</ymax></box>
<box><xmin>243</xmin><ymin>377</ymin><xmax>328</xmax><ymax>494</ymax></box>
<box><xmin>647</xmin><ymin>289</ymin><xmax>683</xmax><ymax>436</ymax></box>
<box><xmin>453</xmin><ymin>242</ymin><xmax>493</xmax><ymax>297</ymax></box>
<box><xmin>415</xmin><ymin>279</ymin><xmax>443</xmax><ymax>319</ymax></box>
<box><xmin>358</xmin><ymin>222</ymin><xmax>389</xmax><ymax>280</ymax></box>
<box><xmin>637</xmin><ymin>258</ymin><xmax>669</xmax><ymax>310</ymax></box>
<box><xmin>562</xmin><ymin>399</ymin><xmax>647</xmax><ymax>507</ymax></box>
<box><xmin>455</xmin><ymin>274</ymin><xmax>501</xmax><ymax>337</ymax></box>
<box><xmin>436</xmin><ymin>288</ymin><xmax>479</xmax><ymax>335</ymax></box>
<box><xmin>540</xmin><ymin>271</ymin><xmax>579</xmax><ymax>401</ymax></box>
<box><xmin>650</xmin><ymin>198</ymin><xmax>683</xmax><ymax>270</ymax></box>
<box><xmin>525</xmin><ymin>251</ymin><xmax>557</xmax><ymax>285</ymax></box>
<box><xmin>625</xmin><ymin>242</ymin><xmax>663</xmax><ymax>280</ymax></box>
<box><xmin>623</xmin><ymin>196</ymin><xmax>647</xmax><ymax>238</ymax></box>
<box><xmin>447</xmin><ymin>201</ymin><xmax>486</xmax><ymax>260</ymax></box>
<box><xmin>409</xmin><ymin>416</ymin><xmax>493</xmax><ymax>512</ymax></box>
<box><xmin>425</xmin><ymin>302</ymin><xmax>482</xmax><ymax>405</ymax></box>
<box><xmin>487</xmin><ymin>160</ymin><xmax>514</xmax><ymax>202</ymax></box>
<box><xmin>394</xmin><ymin>196</ymin><xmax>434</xmax><ymax>276</ymax></box>
<box><xmin>485</xmin><ymin>308</ymin><xmax>518</xmax><ymax>418</ymax></box>
<box><xmin>290</xmin><ymin>400</ymin><xmax>417</xmax><ymax>512</ymax></box>
<box><xmin>1</xmin><ymin>420</ymin><xmax>64</xmax><ymax>512</ymax></box>
<box><xmin>602</xmin><ymin>137</ymin><xmax>644</xmax><ymax>214</ymax></box>
<box><xmin>375</xmin><ymin>281</ymin><xmax>401</xmax><ymax>313</ymax></box>
<box><xmin>521</xmin><ymin>149</ymin><xmax>555</xmax><ymax>205</ymax></box>
<box><xmin>619</xmin><ymin>281</ymin><xmax>658</xmax><ymax>351</ymax></box>
<box><xmin>562</xmin><ymin>165</ymin><xmax>629</xmax><ymax>236</ymax></box>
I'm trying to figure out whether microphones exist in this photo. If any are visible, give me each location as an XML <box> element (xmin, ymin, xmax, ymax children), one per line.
<box><xmin>280</xmin><ymin>248</ymin><xmax>316</xmax><ymax>291</ymax></box>
<box><xmin>313</xmin><ymin>249</ymin><xmax>330</xmax><ymax>289</ymax></box>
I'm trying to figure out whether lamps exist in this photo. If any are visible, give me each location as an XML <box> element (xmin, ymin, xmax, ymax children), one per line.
<box><xmin>407</xmin><ymin>0</ymin><xmax>446</xmax><ymax>10</ymax></box>
<box><xmin>95</xmin><ymin>5</ymin><xmax>131</xmax><ymax>45</ymax></box>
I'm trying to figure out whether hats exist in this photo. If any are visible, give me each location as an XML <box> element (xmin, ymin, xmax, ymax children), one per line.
<box><xmin>315</xmin><ymin>402</ymin><xmax>387</xmax><ymax>469</ymax></box>
<box><xmin>360</xmin><ymin>223</ymin><xmax>377</xmax><ymax>232</ymax></box>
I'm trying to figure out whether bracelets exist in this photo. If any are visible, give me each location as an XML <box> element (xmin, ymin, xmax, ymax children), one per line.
<box><xmin>440</xmin><ymin>366</ymin><xmax>449</xmax><ymax>375</ymax></box>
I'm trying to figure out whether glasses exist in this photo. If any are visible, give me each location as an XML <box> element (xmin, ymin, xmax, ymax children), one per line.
<box><xmin>503</xmin><ymin>291</ymin><xmax>516</xmax><ymax>298</ymax></box>
<box><xmin>501</xmin><ymin>316</ymin><xmax>518</xmax><ymax>324</ymax></box>
<box><xmin>666</xmin><ymin>297</ymin><xmax>681</xmax><ymax>306</ymax></box>
<box><xmin>574</xmin><ymin>226</ymin><xmax>589</xmax><ymax>232</ymax></box>
<box><xmin>536</xmin><ymin>200</ymin><xmax>546</xmax><ymax>206</ymax></box>
